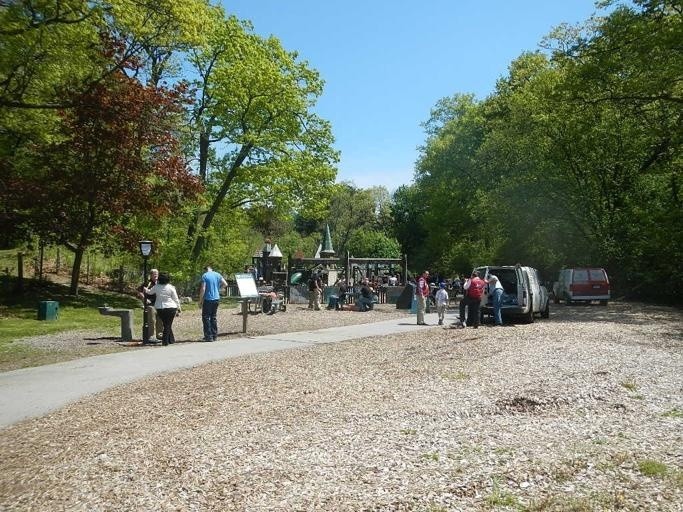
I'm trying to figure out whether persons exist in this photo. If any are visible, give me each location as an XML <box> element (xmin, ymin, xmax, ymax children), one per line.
<box><xmin>144</xmin><ymin>272</ymin><xmax>181</xmax><ymax>345</ymax></box>
<box><xmin>137</xmin><ymin>269</ymin><xmax>164</xmax><ymax>342</ymax></box>
<box><xmin>415</xmin><ymin>270</ymin><xmax>431</xmax><ymax>325</ymax></box>
<box><xmin>485</xmin><ymin>273</ymin><xmax>504</xmax><ymax>326</ymax></box>
<box><xmin>456</xmin><ymin>273</ymin><xmax>486</xmax><ymax>326</ymax></box>
<box><xmin>308</xmin><ymin>271</ymin><xmax>401</xmax><ymax>312</ymax></box>
<box><xmin>429</xmin><ymin>275</ymin><xmax>467</xmax><ymax>325</ymax></box>
<box><xmin>198</xmin><ymin>266</ymin><xmax>228</xmax><ymax>342</ymax></box>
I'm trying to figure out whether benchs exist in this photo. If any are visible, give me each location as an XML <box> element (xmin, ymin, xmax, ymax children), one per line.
<box><xmin>329</xmin><ymin>290</ymin><xmax>379</xmax><ymax>311</ymax></box>
<box><xmin>239</xmin><ymin>286</ymin><xmax>287</xmax><ymax>315</ymax></box>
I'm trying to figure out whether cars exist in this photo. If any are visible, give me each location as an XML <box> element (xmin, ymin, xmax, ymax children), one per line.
<box><xmin>470</xmin><ymin>262</ymin><xmax>611</xmax><ymax>322</ymax></box>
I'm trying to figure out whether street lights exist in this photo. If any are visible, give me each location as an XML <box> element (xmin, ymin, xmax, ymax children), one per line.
<box><xmin>138</xmin><ymin>240</ymin><xmax>155</xmax><ymax>344</ymax></box>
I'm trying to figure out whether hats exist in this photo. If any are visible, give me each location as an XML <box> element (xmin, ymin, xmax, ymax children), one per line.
<box><xmin>440</xmin><ymin>283</ymin><xmax>446</xmax><ymax>288</ymax></box>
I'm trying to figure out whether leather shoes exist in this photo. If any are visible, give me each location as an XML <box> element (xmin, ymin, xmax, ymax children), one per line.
<box><xmin>199</xmin><ymin>338</ymin><xmax>217</xmax><ymax>342</ymax></box>
